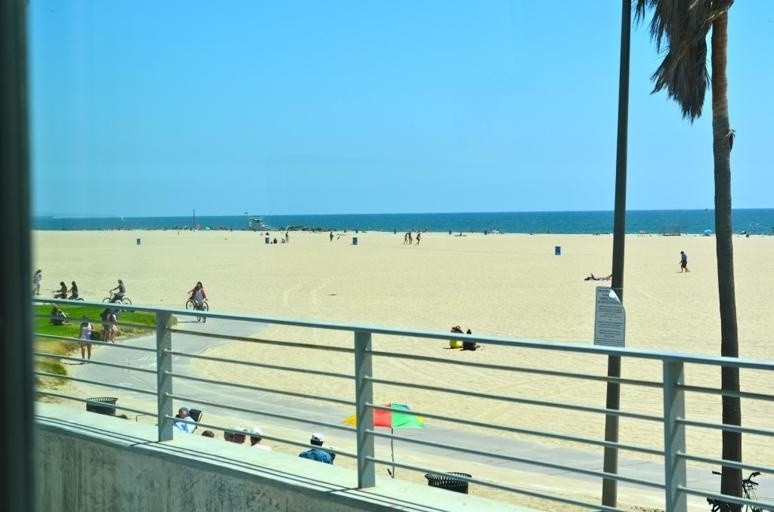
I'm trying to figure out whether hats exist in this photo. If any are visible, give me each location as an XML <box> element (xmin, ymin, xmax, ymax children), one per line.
<box><xmin>234</xmin><ymin>427</ymin><xmax>248</xmax><ymax>435</ymax></box>
<box><xmin>251</xmin><ymin>427</ymin><xmax>263</xmax><ymax>440</ymax></box>
<box><xmin>311</xmin><ymin>433</ymin><xmax>324</xmax><ymax>442</ymax></box>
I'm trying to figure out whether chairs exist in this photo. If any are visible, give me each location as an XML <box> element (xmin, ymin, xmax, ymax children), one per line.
<box><xmin>188</xmin><ymin>408</ymin><xmax>203</xmax><ymax>430</ymax></box>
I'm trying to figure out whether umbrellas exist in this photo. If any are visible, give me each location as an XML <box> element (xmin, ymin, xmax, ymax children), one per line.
<box><xmin>344</xmin><ymin>402</ymin><xmax>426</xmax><ymax>478</ymax></box>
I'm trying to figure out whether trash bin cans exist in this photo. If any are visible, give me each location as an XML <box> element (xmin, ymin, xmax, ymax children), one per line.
<box><xmin>85</xmin><ymin>397</ymin><xmax>118</xmax><ymax>416</ymax></box>
<box><xmin>556</xmin><ymin>246</ymin><xmax>560</xmax><ymax>255</ymax></box>
<box><xmin>353</xmin><ymin>237</ymin><xmax>357</xmax><ymax>245</ymax></box>
<box><xmin>425</xmin><ymin>472</ymin><xmax>472</xmax><ymax>494</ymax></box>
<box><xmin>137</xmin><ymin>239</ymin><xmax>140</xmax><ymax>244</ymax></box>
<box><xmin>265</xmin><ymin>237</ymin><xmax>269</xmax><ymax>244</ymax></box>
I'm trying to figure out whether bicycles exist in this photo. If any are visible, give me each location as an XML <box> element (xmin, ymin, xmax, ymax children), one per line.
<box><xmin>191</xmin><ymin>300</ymin><xmax>207</xmax><ymax>323</ymax></box>
<box><xmin>52</xmin><ymin>289</ymin><xmax>83</xmax><ymax>303</ymax></box>
<box><xmin>102</xmin><ymin>289</ymin><xmax>133</xmax><ymax>311</ymax></box>
<box><xmin>185</xmin><ymin>296</ymin><xmax>209</xmax><ymax>312</ymax></box>
<box><xmin>708</xmin><ymin>468</ymin><xmax>764</xmax><ymax>512</ymax></box>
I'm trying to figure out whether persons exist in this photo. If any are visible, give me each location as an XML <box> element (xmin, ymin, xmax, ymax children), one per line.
<box><xmin>679</xmin><ymin>251</ymin><xmax>690</xmax><ymax>273</ymax></box>
<box><xmin>584</xmin><ymin>273</ymin><xmax>613</xmax><ymax>281</ymax></box>
<box><xmin>32</xmin><ymin>269</ymin><xmax>127</xmax><ymax>363</ymax></box>
<box><xmin>298</xmin><ymin>433</ymin><xmax>334</xmax><ymax>464</ymax></box>
<box><xmin>172</xmin><ymin>407</ymin><xmax>196</xmax><ymax>434</ymax></box>
<box><xmin>201</xmin><ymin>427</ymin><xmax>272</xmax><ymax>452</ymax></box>
<box><xmin>450</xmin><ymin>325</ymin><xmax>463</xmax><ymax>348</ymax></box>
<box><xmin>463</xmin><ymin>329</ymin><xmax>480</xmax><ymax>351</ymax></box>
<box><xmin>187</xmin><ymin>282</ymin><xmax>208</xmax><ymax>307</ymax></box>
